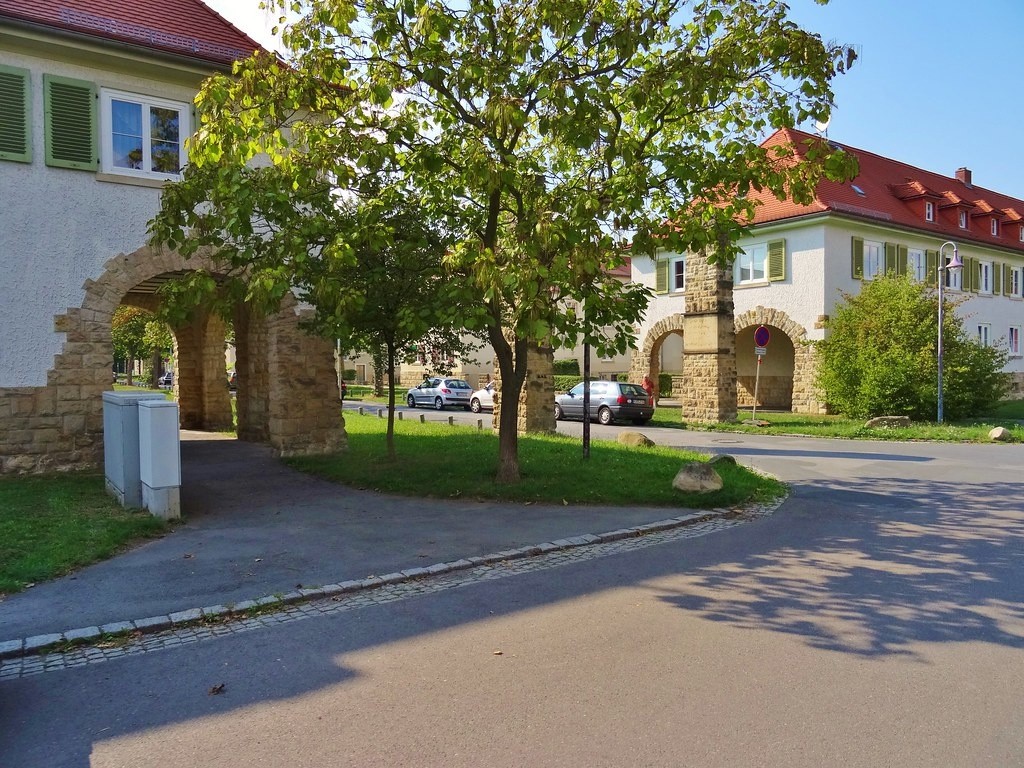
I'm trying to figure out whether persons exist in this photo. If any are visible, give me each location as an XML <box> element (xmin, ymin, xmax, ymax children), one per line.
<box><xmin>640</xmin><ymin>374</ymin><xmax>655</xmax><ymax>396</ymax></box>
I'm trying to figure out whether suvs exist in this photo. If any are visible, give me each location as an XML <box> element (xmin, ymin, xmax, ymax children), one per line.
<box><xmin>554</xmin><ymin>381</ymin><xmax>654</xmax><ymax>426</ymax></box>
<box><xmin>405</xmin><ymin>376</ymin><xmax>474</xmax><ymax>412</ymax></box>
<box><xmin>163</xmin><ymin>373</ymin><xmax>174</xmax><ymax>385</ymax></box>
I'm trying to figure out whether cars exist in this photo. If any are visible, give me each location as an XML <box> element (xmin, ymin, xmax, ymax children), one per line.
<box><xmin>470</xmin><ymin>380</ymin><xmax>496</xmax><ymax>413</ymax></box>
<box><xmin>228</xmin><ymin>372</ymin><xmax>237</xmax><ymax>391</ymax></box>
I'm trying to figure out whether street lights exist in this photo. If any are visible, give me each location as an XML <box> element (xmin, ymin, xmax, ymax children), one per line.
<box><xmin>936</xmin><ymin>241</ymin><xmax>966</xmax><ymax>426</ymax></box>
